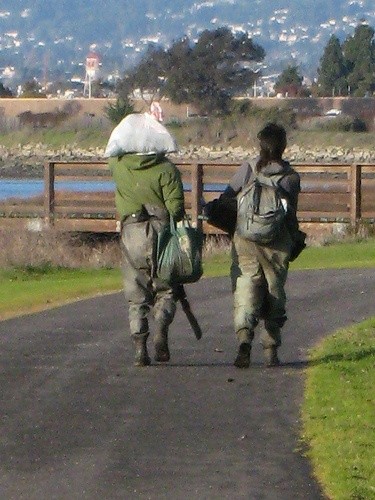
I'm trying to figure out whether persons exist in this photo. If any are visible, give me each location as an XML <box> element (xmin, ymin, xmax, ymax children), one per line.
<box><xmin>104</xmin><ymin>106</ymin><xmax>185</xmax><ymax>365</ymax></box>
<box><xmin>221</xmin><ymin>122</ymin><xmax>299</xmax><ymax>366</ymax></box>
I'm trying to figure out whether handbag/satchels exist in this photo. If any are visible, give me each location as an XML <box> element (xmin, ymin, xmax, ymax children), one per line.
<box><xmin>157</xmin><ymin>209</ymin><xmax>204</xmax><ymax>283</ymax></box>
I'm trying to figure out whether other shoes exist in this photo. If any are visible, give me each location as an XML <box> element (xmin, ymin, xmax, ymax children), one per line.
<box><xmin>234</xmin><ymin>328</ymin><xmax>254</xmax><ymax>369</ymax></box>
<box><xmin>133</xmin><ymin>337</ymin><xmax>151</xmax><ymax>366</ymax></box>
<box><xmin>264</xmin><ymin>347</ymin><xmax>278</xmax><ymax>367</ymax></box>
<box><xmin>153</xmin><ymin>319</ymin><xmax>169</xmax><ymax>362</ymax></box>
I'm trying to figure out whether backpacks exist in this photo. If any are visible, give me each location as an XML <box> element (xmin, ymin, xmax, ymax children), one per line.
<box><xmin>234</xmin><ymin>159</ymin><xmax>299</xmax><ymax>243</ymax></box>
